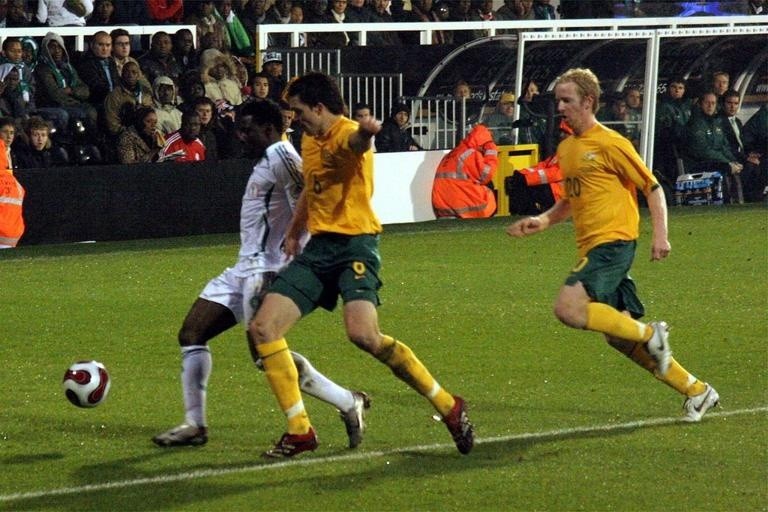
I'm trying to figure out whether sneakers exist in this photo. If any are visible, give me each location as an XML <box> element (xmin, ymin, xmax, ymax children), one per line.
<box><xmin>674</xmin><ymin>382</ymin><xmax>723</xmax><ymax>427</ymax></box>
<box><xmin>335</xmin><ymin>389</ymin><xmax>372</xmax><ymax>449</ymax></box>
<box><xmin>150</xmin><ymin>422</ymin><xmax>210</xmax><ymax>448</ymax></box>
<box><xmin>642</xmin><ymin>320</ymin><xmax>672</xmax><ymax>378</ymax></box>
<box><xmin>438</xmin><ymin>396</ymin><xmax>476</xmax><ymax>455</ymax></box>
<box><xmin>260</xmin><ymin>426</ymin><xmax>320</xmax><ymax>464</ymax></box>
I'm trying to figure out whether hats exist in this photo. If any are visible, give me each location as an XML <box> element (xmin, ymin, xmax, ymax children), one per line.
<box><xmin>391</xmin><ymin>102</ymin><xmax>411</xmax><ymax>125</ymax></box>
<box><xmin>261</xmin><ymin>51</ymin><xmax>285</xmax><ymax>68</ymax></box>
<box><xmin>500</xmin><ymin>93</ymin><xmax>514</xmax><ymax>103</ymax></box>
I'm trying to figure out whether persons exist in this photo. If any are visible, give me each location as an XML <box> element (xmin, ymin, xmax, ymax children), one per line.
<box><xmin>508</xmin><ymin>67</ymin><xmax>720</xmax><ymax>423</ymax></box>
<box><xmin>152</xmin><ymin>101</ymin><xmax>371</xmax><ymax>449</ymax></box>
<box><xmin>246</xmin><ymin>71</ymin><xmax>474</xmax><ymax>456</ymax></box>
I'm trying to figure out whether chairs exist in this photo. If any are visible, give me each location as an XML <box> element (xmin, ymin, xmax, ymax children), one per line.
<box><xmin>634</xmin><ymin>131</ymin><xmax>745</xmax><ymax>208</ymax></box>
<box><xmin>1</xmin><ymin>45</ymin><xmax>256</xmax><ymax>167</ymax></box>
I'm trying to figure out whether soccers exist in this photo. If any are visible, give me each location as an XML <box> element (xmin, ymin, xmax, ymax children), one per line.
<box><xmin>63</xmin><ymin>361</ymin><xmax>111</xmax><ymax>407</ymax></box>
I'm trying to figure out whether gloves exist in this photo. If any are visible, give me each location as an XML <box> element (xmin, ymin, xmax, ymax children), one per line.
<box><xmin>504</xmin><ymin>170</ymin><xmax>528</xmax><ymax>195</ymax></box>
<box><xmin>484</xmin><ymin>141</ymin><xmax>498</xmax><ymax>155</ymax></box>
